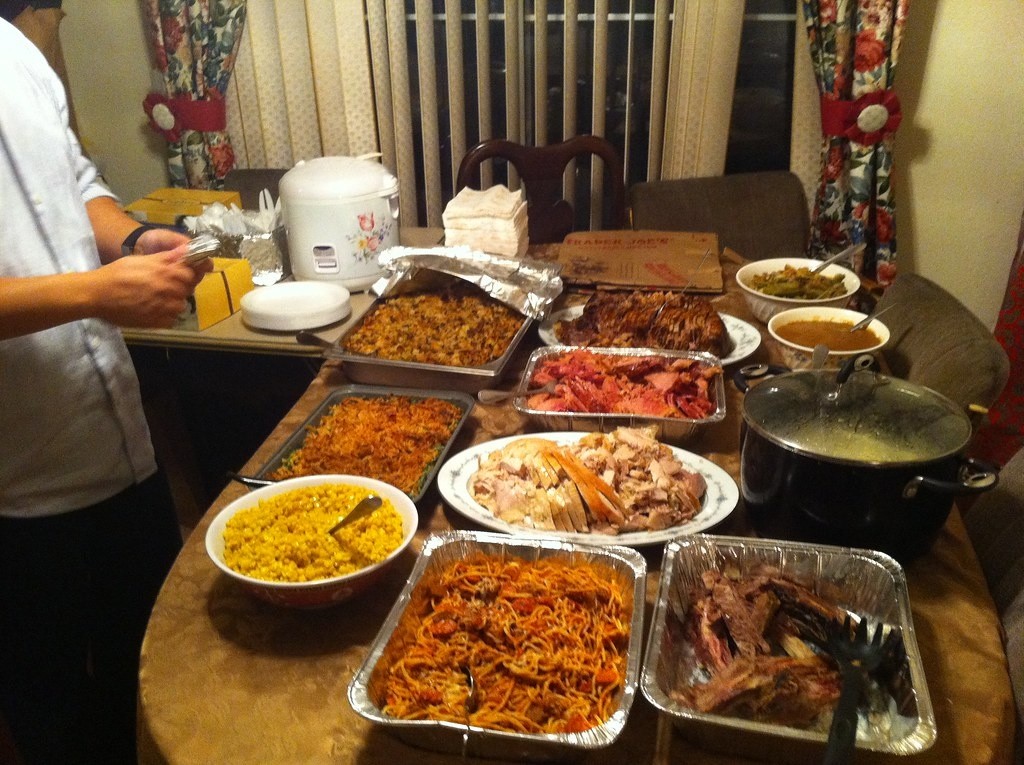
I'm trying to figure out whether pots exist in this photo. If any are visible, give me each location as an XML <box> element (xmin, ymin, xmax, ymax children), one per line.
<box><xmin>729</xmin><ymin>356</ymin><xmax>999</xmax><ymax>565</ymax></box>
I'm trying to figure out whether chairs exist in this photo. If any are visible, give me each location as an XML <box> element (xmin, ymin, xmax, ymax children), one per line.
<box><xmin>455</xmin><ymin>136</ymin><xmax>630</xmax><ymax>247</ymax></box>
<box><xmin>870</xmin><ymin>270</ymin><xmax>1009</xmax><ymax>420</ymax></box>
<box><xmin>632</xmin><ymin>173</ymin><xmax>809</xmax><ymax>260</ymax></box>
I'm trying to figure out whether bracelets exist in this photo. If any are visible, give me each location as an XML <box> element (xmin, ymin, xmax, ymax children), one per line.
<box><xmin>121</xmin><ymin>226</ymin><xmax>156</xmax><ymax>256</ymax></box>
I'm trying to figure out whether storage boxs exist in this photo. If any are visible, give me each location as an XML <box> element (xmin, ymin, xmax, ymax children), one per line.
<box><xmin>124</xmin><ymin>189</ymin><xmax>243</xmax><ymax>236</ymax></box>
<box><xmin>176</xmin><ymin>257</ymin><xmax>253</xmax><ymax>331</ymax></box>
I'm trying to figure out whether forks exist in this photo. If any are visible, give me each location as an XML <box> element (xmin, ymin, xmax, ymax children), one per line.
<box><xmin>823</xmin><ymin>614</ymin><xmax>896</xmax><ymax>765</ymax></box>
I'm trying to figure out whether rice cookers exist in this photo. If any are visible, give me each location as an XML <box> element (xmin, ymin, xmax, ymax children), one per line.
<box><xmin>278</xmin><ymin>157</ymin><xmax>404</xmax><ymax>293</ymax></box>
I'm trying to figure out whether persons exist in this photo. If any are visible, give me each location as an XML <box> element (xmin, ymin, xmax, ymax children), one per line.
<box><xmin>1</xmin><ymin>16</ymin><xmax>213</xmax><ymax>765</ymax></box>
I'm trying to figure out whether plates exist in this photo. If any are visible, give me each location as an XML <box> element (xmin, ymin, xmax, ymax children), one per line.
<box><xmin>241</xmin><ymin>281</ymin><xmax>352</xmax><ymax>331</ymax></box>
<box><xmin>538</xmin><ymin>303</ymin><xmax>762</xmax><ymax>369</ymax></box>
<box><xmin>437</xmin><ymin>430</ymin><xmax>739</xmax><ymax>546</ymax></box>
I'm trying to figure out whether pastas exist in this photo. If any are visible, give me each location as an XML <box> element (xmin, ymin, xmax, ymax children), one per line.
<box><xmin>382</xmin><ymin>559</ymin><xmax>629</xmax><ymax>734</ymax></box>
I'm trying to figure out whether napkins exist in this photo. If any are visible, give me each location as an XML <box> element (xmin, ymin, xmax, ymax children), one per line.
<box><xmin>443</xmin><ymin>185</ymin><xmax>529</xmax><ymax>259</ymax></box>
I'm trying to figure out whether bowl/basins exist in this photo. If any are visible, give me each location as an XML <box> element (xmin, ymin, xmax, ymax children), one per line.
<box><xmin>735</xmin><ymin>256</ymin><xmax>861</xmax><ymax>325</ymax></box>
<box><xmin>205</xmin><ymin>475</ymin><xmax>418</xmax><ymax>609</ymax></box>
<box><xmin>768</xmin><ymin>306</ymin><xmax>890</xmax><ymax>369</ymax></box>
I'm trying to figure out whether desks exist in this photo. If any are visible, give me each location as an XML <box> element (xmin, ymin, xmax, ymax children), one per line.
<box><xmin>120</xmin><ymin>227</ymin><xmax>1024</xmax><ymax>765</ymax></box>
